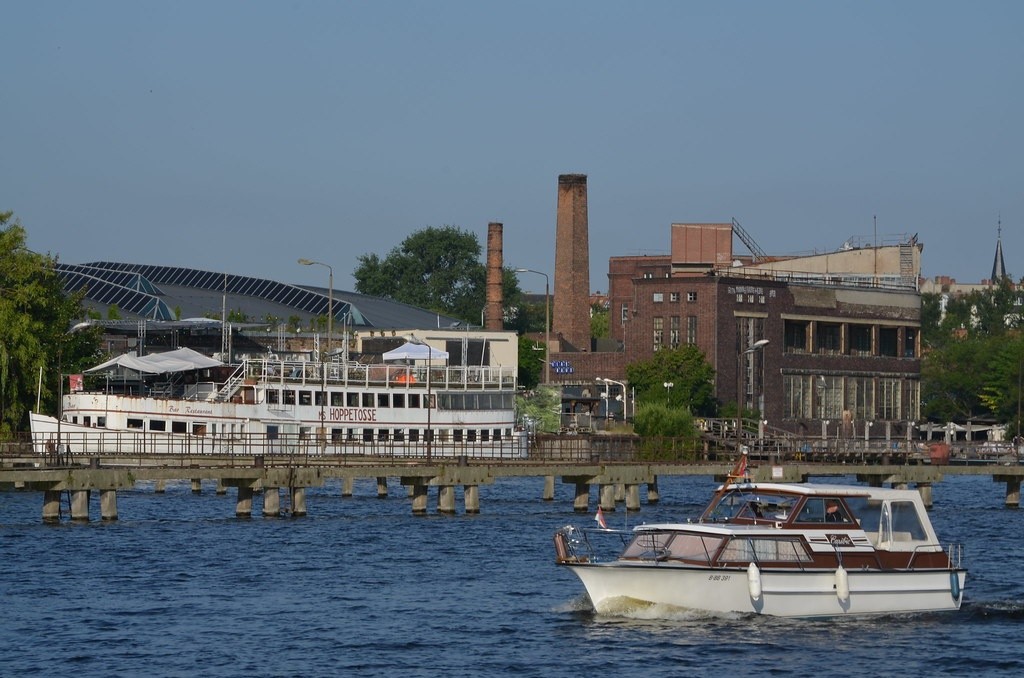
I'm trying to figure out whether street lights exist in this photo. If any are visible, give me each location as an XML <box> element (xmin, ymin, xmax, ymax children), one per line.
<box><xmin>737</xmin><ymin>339</ymin><xmax>771</xmax><ymax>445</ymax></box>
<box><xmin>604</xmin><ymin>379</ymin><xmax>627</xmax><ymax>421</ymax></box>
<box><xmin>516</xmin><ymin>268</ymin><xmax>550</xmax><ymax>386</ymax></box>
<box><xmin>58</xmin><ymin>322</ymin><xmax>92</xmax><ymax>464</ymax></box>
<box><xmin>298</xmin><ymin>258</ymin><xmax>333</xmax><ymax>455</ymax></box>
<box><xmin>401</xmin><ymin>336</ymin><xmax>431</xmax><ymax>466</ymax></box>
<box><xmin>595</xmin><ymin>377</ymin><xmax>608</xmax><ymax>430</ymax></box>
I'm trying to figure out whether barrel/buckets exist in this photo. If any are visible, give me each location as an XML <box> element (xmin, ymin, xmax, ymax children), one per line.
<box><xmin>929</xmin><ymin>444</ymin><xmax>950</xmax><ymax>465</ymax></box>
<box><xmin>893</xmin><ymin>443</ymin><xmax>896</xmax><ymax>448</ymax></box>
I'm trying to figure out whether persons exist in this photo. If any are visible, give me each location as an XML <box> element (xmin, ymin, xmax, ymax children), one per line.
<box><xmin>808</xmin><ymin>501</ymin><xmax>842</xmax><ymax>522</ymax></box>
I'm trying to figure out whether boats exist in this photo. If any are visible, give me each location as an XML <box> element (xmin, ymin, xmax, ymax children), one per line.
<box><xmin>26</xmin><ymin>331</ymin><xmax>531</xmax><ymax>457</ymax></box>
<box><xmin>550</xmin><ymin>447</ymin><xmax>969</xmax><ymax>620</ymax></box>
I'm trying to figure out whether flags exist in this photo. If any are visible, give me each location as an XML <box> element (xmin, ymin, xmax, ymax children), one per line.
<box><xmin>740</xmin><ymin>457</ymin><xmax>747</xmax><ymax>477</ymax></box>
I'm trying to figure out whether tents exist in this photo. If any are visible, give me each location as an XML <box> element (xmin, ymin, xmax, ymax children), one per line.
<box><xmin>382</xmin><ymin>342</ymin><xmax>449</xmax><ymax>366</ymax></box>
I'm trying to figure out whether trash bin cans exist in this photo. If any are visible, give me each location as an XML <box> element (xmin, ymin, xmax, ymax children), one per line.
<box><xmin>927</xmin><ymin>442</ymin><xmax>952</xmax><ymax>465</ymax></box>
